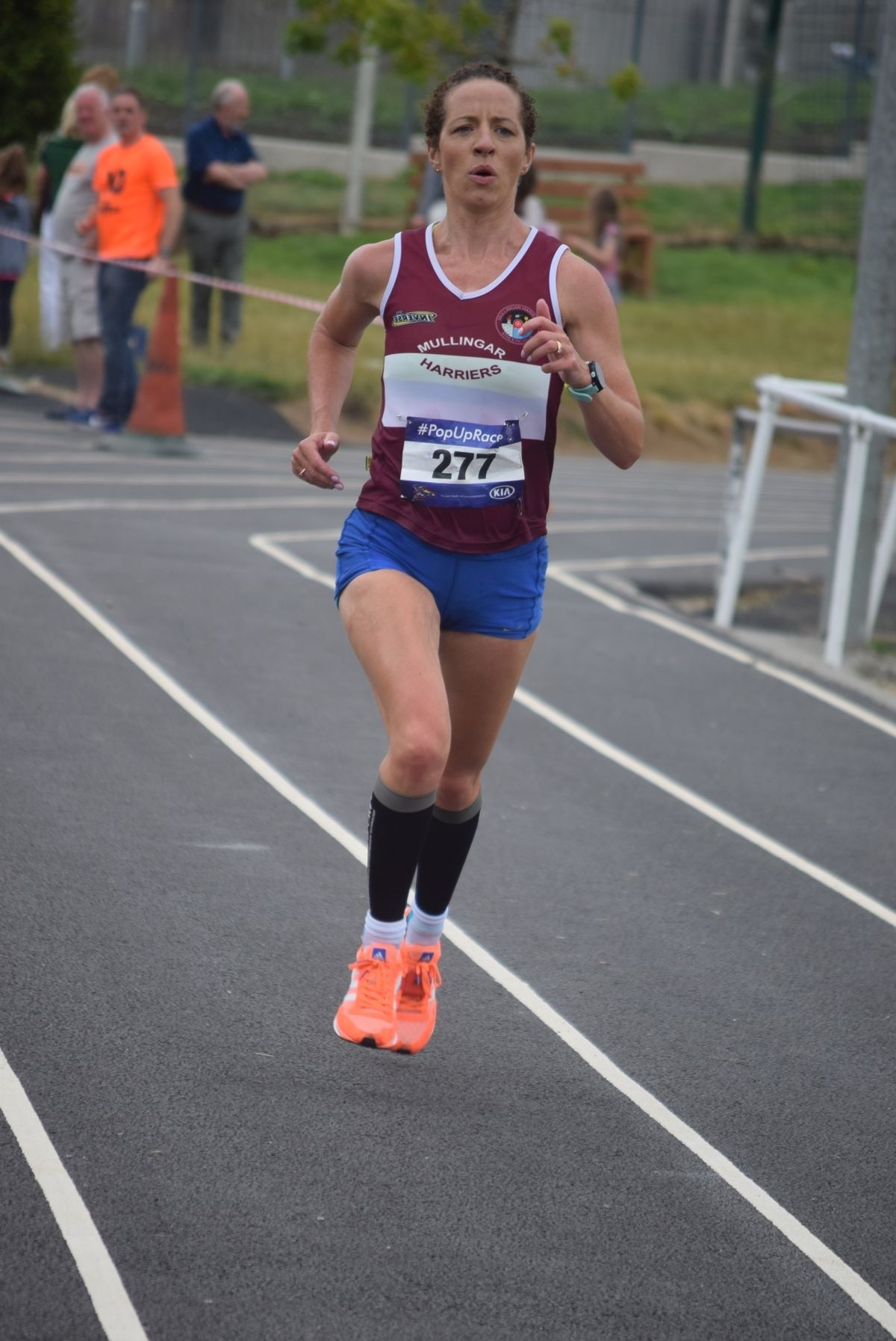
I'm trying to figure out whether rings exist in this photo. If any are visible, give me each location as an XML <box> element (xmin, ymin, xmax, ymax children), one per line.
<box><xmin>554</xmin><ymin>340</ymin><xmax>562</xmax><ymax>354</ymax></box>
<box><xmin>299</xmin><ymin>468</ymin><xmax>306</xmax><ymax>476</ymax></box>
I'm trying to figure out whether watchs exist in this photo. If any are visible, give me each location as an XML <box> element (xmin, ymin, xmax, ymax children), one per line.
<box><xmin>565</xmin><ymin>361</ymin><xmax>606</xmax><ymax>402</ymax></box>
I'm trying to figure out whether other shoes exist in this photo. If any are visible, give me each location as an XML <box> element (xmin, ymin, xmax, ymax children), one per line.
<box><xmin>77</xmin><ymin>410</ymin><xmax>124</xmax><ymax>433</ymax></box>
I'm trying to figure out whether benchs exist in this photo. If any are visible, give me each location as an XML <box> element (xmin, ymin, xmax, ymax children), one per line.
<box><xmin>403</xmin><ymin>146</ymin><xmax>657</xmax><ymax>297</ymax></box>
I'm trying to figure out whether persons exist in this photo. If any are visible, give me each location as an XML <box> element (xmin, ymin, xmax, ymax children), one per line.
<box><xmin>292</xmin><ymin>61</ymin><xmax>644</xmax><ymax>1059</ymax></box>
<box><xmin>409</xmin><ymin>150</ymin><xmax>445</xmax><ymax>229</ymax></box>
<box><xmin>181</xmin><ymin>76</ymin><xmax>266</xmax><ymax>352</ymax></box>
<box><xmin>562</xmin><ymin>189</ymin><xmax>619</xmax><ymax>304</ymax></box>
<box><xmin>74</xmin><ymin>87</ymin><xmax>184</xmax><ymax>434</ymax></box>
<box><xmin>514</xmin><ymin>162</ymin><xmax>544</xmax><ymax>227</ymax></box>
<box><xmin>44</xmin><ymin>82</ymin><xmax>120</xmax><ymax>424</ymax></box>
<box><xmin>33</xmin><ymin>63</ymin><xmax>122</xmax><ymax>354</ymax></box>
<box><xmin>0</xmin><ymin>142</ymin><xmax>30</xmax><ymax>393</ymax></box>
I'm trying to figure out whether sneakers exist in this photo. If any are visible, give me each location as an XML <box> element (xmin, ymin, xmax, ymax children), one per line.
<box><xmin>389</xmin><ymin>906</ymin><xmax>444</xmax><ymax>1053</ymax></box>
<box><xmin>333</xmin><ymin>943</ymin><xmax>402</xmax><ymax>1046</ymax></box>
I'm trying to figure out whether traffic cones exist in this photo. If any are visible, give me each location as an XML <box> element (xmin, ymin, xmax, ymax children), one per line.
<box><xmin>96</xmin><ymin>259</ymin><xmax>198</xmax><ymax>455</ymax></box>
